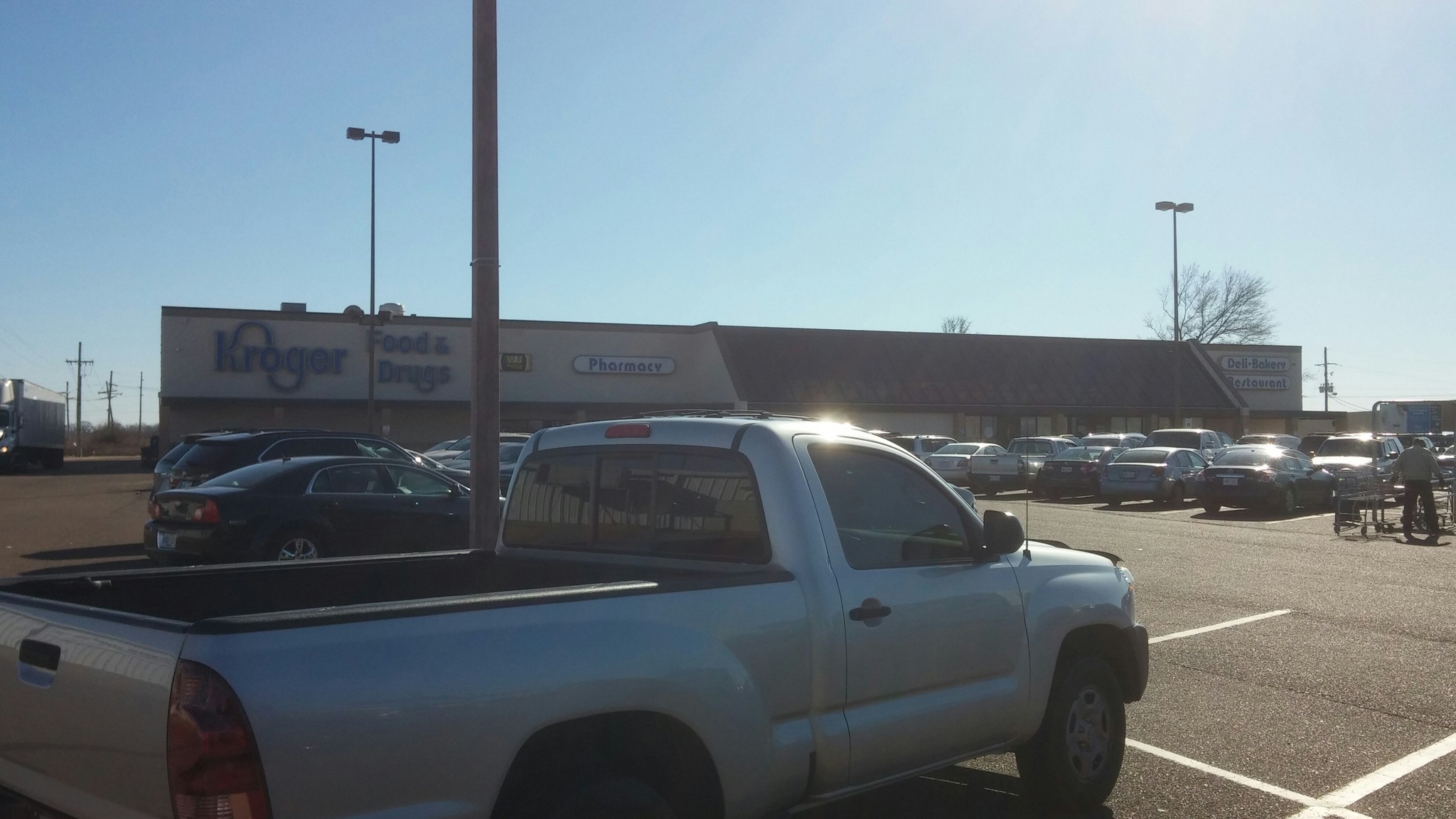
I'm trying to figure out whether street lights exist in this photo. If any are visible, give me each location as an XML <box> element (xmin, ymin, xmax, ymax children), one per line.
<box><xmin>1155</xmin><ymin>201</ymin><xmax>1194</xmax><ymax>428</ymax></box>
<box><xmin>345</xmin><ymin>126</ymin><xmax>400</xmax><ymax>435</ymax></box>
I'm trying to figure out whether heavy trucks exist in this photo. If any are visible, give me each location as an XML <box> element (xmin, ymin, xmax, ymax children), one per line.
<box><xmin>0</xmin><ymin>378</ymin><xmax>66</xmax><ymax>476</ymax></box>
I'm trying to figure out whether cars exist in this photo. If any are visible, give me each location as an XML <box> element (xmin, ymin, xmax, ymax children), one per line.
<box><xmin>1400</xmin><ymin>437</ymin><xmax>1454</xmax><ymax>478</ymax></box>
<box><xmin>1238</xmin><ymin>432</ymin><xmax>1301</xmax><ymax>451</ymax></box>
<box><xmin>925</xmin><ymin>442</ymin><xmax>1008</xmax><ymax>494</ymax></box>
<box><xmin>1215</xmin><ymin>431</ymin><xmax>1233</xmax><ymax>448</ymax></box>
<box><xmin>1099</xmin><ymin>446</ymin><xmax>1207</xmax><ymax>507</ymax></box>
<box><xmin>1041</xmin><ymin>446</ymin><xmax>1130</xmax><ymax>499</ymax></box>
<box><xmin>1209</xmin><ymin>443</ymin><xmax>1290</xmax><ymax>465</ymax></box>
<box><xmin>144</xmin><ymin>426</ymin><xmax>530</xmax><ymax>565</ymax></box>
<box><xmin>1194</xmin><ymin>444</ymin><xmax>1339</xmax><ymax>517</ymax></box>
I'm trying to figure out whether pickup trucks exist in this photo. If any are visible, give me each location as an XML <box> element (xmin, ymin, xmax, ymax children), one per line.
<box><xmin>968</xmin><ymin>436</ymin><xmax>1079</xmax><ymax>497</ymax></box>
<box><xmin>1141</xmin><ymin>428</ymin><xmax>1224</xmax><ymax>463</ymax></box>
<box><xmin>0</xmin><ymin>407</ymin><xmax>1153</xmax><ymax>819</ymax></box>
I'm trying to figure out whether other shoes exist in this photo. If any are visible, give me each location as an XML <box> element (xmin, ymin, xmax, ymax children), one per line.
<box><xmin>1427</xmin><ymin>528</ymin><xmax>1444</xmax><ymax>535</ymax></box>
<box><xmin>1403</xmin><ymin>532</ymin><xmax>1412</xmax><ymax>535</ymax></box>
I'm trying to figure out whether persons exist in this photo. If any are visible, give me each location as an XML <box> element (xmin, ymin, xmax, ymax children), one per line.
<box><xmin>1389</xmin><ymin>438</ymin><xmax>1445</xmax><ymax>533</ymax></box>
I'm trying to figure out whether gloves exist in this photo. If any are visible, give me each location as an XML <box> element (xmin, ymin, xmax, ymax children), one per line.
<box><xmin>1390</xmin><ymin>478</ymin><xmax>1398</xmax><ymax>484</ymax></box>
<box><xmin>1439</xmin><ymin>478</ymin><xmax>1445</xmax><ymax>486</ymax></box>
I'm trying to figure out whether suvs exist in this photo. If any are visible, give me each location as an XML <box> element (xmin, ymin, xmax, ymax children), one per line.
<box><xmin>1075</xmin><ymin>432</ymin><xmax>1148</xmax><ymax>449</ymax></box>
<box><xmin>1297</xmin><ymin>432</ymin><xmax>1334</xmax><ymax>459</ymax></box>
<box><xmin>1312</xmin><ymin>431</ymin><xmax>1406</xmax><ymax>509</ymax></box>
<box><xmin>1036</xmin><ymin>434</ymin><xmax>1081</xmax><ymax>444</ymax></box>
<box><xmin>888</xmin><ymin>434</ymin><xmax>960</xmax><ymax>464</ymax></box>
